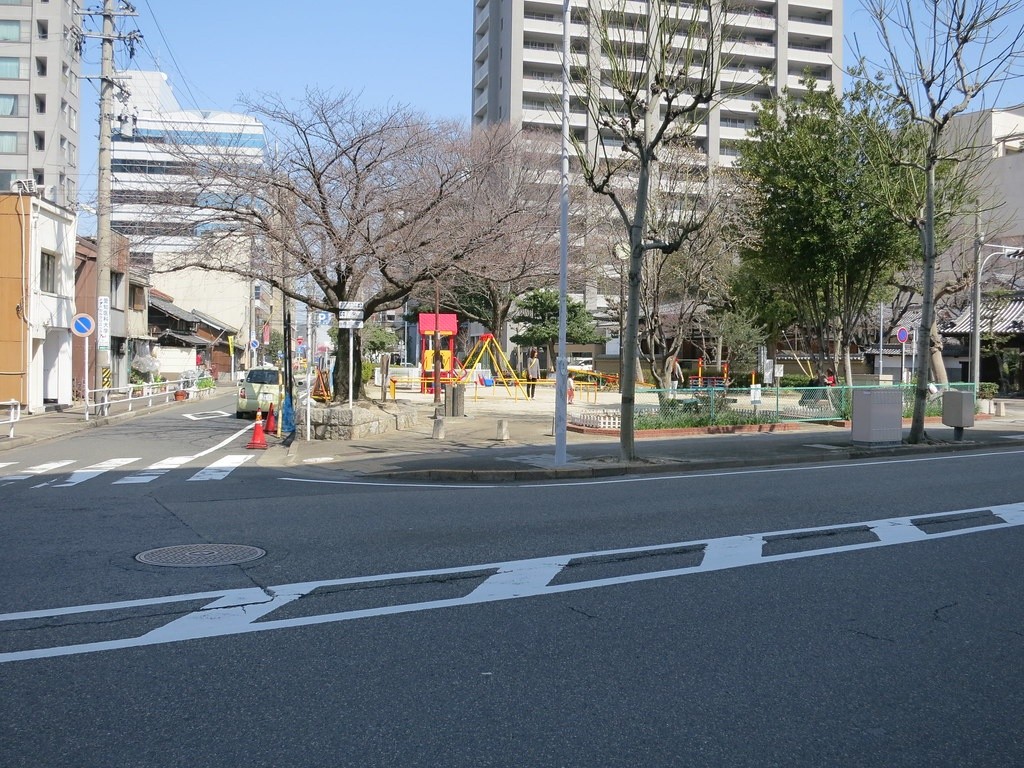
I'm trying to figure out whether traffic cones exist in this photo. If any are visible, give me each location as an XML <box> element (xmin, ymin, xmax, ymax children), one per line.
<box><xmin>263</xmin><ymin>402</ymin><xmax>278</xmax><ymax>434</ymax></box>
<box><xmin>246</xmin><ymin>408</ymin><xmax>269</xmax><ymax>450</ymax></box>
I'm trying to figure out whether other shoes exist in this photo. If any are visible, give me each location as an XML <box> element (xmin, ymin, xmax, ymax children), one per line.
<box><xmin>528</xmin><ymin>397</ymin><xmax>536</xmax><ymax>400</ymax></box>
<box><xmin>569</xmin><ymin>401</ymin><xmax>574</xmax><ymax>404</ymax></box>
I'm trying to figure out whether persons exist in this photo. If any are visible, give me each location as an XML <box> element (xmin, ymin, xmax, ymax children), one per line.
<box><xmin>567</xmin><ymin>372</ymin><xmax>576</xmax><ymax>404</ymax></box>
<box><xmin>823</xmin><ymin>368</ymin><xmax>836</xmax><ymax>410</ymax></box>
<box><xmin>526</xmin><ymin>350</ymin><xmax>540</xmax><ymax>401</ymax></box>
<box><xmin>671</xmin><ymin>359</ymin><xmax>684</xmax><ymax>398</ymax></box>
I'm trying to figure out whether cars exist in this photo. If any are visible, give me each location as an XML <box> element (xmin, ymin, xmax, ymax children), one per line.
<box><xmin>235</xmin><ymin>365</ymin><xmax>303</xmax><ymax>420</ymax></box>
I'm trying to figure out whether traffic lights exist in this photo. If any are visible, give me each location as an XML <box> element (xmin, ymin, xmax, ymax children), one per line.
<box><xmin>1005</xmin><ymin>248</ymin><xmax>1024</xmax><ymax>259</ymax></box>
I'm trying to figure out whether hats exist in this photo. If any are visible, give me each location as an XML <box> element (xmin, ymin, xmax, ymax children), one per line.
<box><xmin>676</xmin><ymin>357</ymin><xmax>679</xmax><ymax>362</ymax></box>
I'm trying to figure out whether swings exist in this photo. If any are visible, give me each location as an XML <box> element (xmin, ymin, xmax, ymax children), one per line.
<box><xmin>478</xmin><ymin>340</ymin><xmax>494</xmax><ymax>386</ymax></box>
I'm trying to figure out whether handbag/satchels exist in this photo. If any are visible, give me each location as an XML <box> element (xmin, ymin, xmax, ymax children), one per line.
<box><xmin>521</xmin><ymin>370</ymin><xmax>528</xmax><ymax>378</ymax></box>
